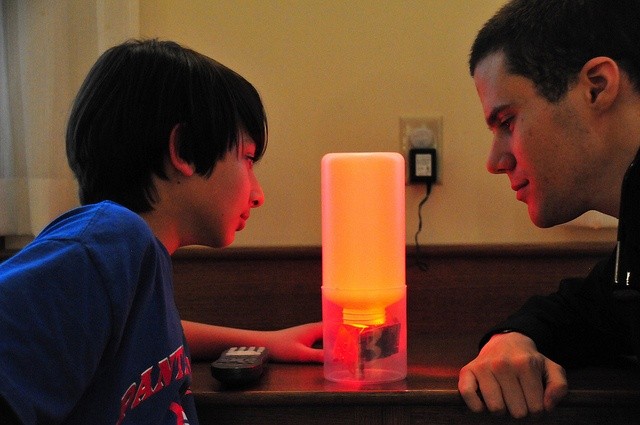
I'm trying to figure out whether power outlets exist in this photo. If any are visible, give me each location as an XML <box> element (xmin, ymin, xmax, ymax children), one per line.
<box><xmin>401</xmin><ymin>116</ymin><xmax>442</xmax><ymax>188</ymax></box>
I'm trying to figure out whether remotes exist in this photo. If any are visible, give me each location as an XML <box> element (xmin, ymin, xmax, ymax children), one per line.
<box><xmin>208</xmin><ymin>345</ymin><xmax>268</xmax><ymax>387</ymax></box>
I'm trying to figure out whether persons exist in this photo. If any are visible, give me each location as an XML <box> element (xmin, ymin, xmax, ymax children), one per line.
<box><xmin>458</xmin><ymin>1</ymin><xmax>640</xmax><ymax>425</ymax></box>
<box><xmin>1</xmin><ymin>37</ymin><xmax>325</xmax><ymax>424</ymax></box>
